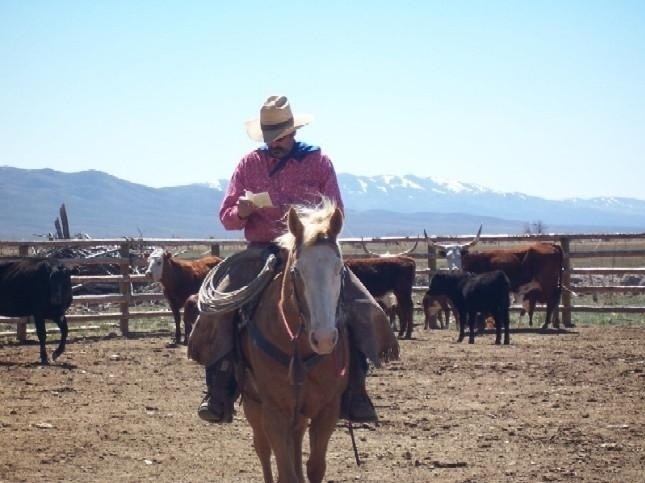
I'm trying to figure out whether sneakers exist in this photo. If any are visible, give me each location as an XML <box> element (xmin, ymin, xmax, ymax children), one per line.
<box><xmin>199</xmin><ymin>400</ymin><xmax>234</xmax><ymax>424</ymax></box>
<box><xmin>343</xmin><ymin>394</ymin><xmax>378</xmax><ymax>421</ymax></box>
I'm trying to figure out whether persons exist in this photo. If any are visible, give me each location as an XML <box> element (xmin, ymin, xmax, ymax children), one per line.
<box><xmin>196</xmin><ymin>94</ymin><xmax>379</xmax><ymax>423</ymax></box>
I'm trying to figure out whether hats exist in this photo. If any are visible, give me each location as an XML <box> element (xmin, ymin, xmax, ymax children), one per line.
<box><xmin>244</xmin><ymin>95</ymin><xmax>314</xmax><ymax>144</ymax></box>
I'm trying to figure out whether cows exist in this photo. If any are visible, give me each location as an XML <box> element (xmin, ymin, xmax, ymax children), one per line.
<box><xmin>144</xmin><ymin>247</ymin><xmax>223</xmax><ymax>345</ymax></box>
<box><xmin>422</xmin><ymin>223</ymin><xmax>578</xmax><ymax>346</ymax></box>
<box><xmin>183</xmin><ymin>293</ymin><xmax>199</xmax><ymax>346</ymax></box>
<box><xmin>0</xmin><ymin>256</ymin><xmax>81</xmax><ymax>365</ymax></box>
<box><xmin>343</xmin><ymin>233</ymin><xmax>420</xmax><ymax>339</ymax></box>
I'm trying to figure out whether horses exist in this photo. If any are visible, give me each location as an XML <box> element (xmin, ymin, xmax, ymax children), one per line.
<box><xmin>233</xmin><ymin>191</ymin><xmax>351</xmax><ymax>483</ymax></box>
